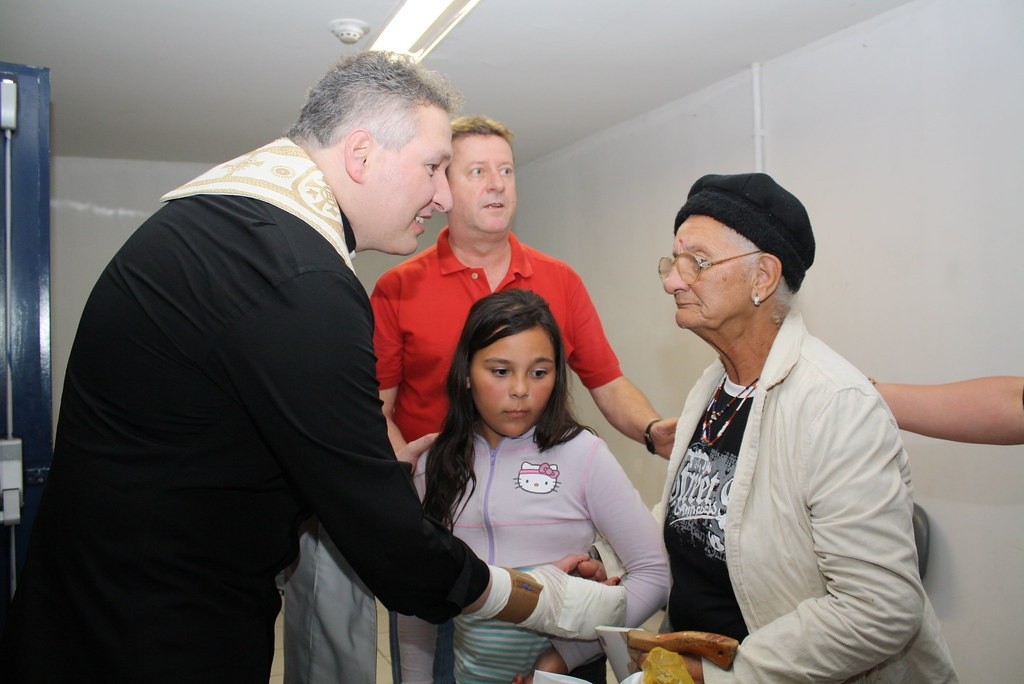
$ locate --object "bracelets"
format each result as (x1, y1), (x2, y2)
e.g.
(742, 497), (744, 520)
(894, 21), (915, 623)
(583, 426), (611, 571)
(644, 420), (660, 454)
(868, 377), (876, 385)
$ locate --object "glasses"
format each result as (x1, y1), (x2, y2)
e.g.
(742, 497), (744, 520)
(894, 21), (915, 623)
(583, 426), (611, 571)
(658, 250), (763, 285)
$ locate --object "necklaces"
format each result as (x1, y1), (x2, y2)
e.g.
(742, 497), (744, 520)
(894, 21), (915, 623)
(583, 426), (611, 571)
(700, 376), (760, 451)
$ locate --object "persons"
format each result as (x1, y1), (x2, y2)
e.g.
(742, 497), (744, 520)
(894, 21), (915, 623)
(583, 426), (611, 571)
(868, 375), (1024, 446)
(409, 287), (669, 684)
(367, 116), (679, 461)
(578, 173), (959, 684)
(0, 50), (627, 684)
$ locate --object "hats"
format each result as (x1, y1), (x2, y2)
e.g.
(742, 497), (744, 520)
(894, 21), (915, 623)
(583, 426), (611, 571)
(673, 172), (816, 294)
